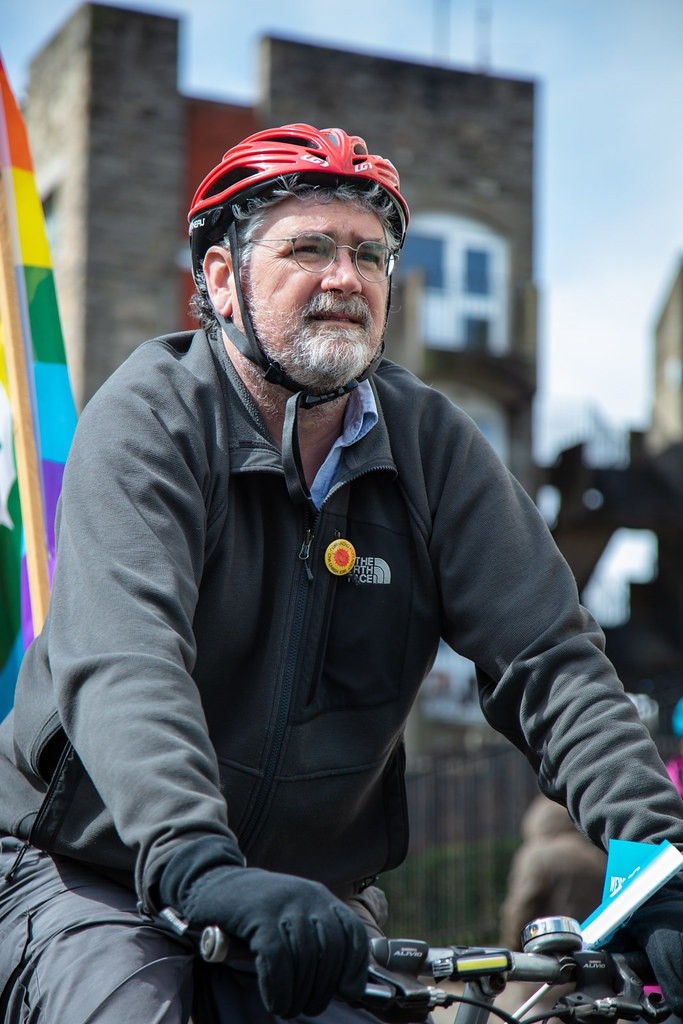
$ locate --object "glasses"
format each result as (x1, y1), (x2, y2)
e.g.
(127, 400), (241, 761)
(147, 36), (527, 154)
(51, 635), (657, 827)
(224, 232), (400, 283)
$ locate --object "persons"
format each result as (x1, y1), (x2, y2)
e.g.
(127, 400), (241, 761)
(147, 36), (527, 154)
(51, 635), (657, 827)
(0, 121), (682, 1023)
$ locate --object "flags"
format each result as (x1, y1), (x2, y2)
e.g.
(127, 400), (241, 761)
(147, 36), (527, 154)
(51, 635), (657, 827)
(0, 53), (81, 725)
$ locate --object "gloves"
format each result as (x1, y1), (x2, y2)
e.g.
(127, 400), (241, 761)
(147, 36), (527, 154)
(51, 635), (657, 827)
(162, 836), (368, 1021)
(622, 891), (683, 1024)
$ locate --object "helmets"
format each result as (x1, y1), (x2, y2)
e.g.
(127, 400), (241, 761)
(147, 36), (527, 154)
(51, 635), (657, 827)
(187, 123), (410, 298)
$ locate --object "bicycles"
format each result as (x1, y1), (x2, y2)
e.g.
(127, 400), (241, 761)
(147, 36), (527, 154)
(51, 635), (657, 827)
(198, 914), (674, 1023)
